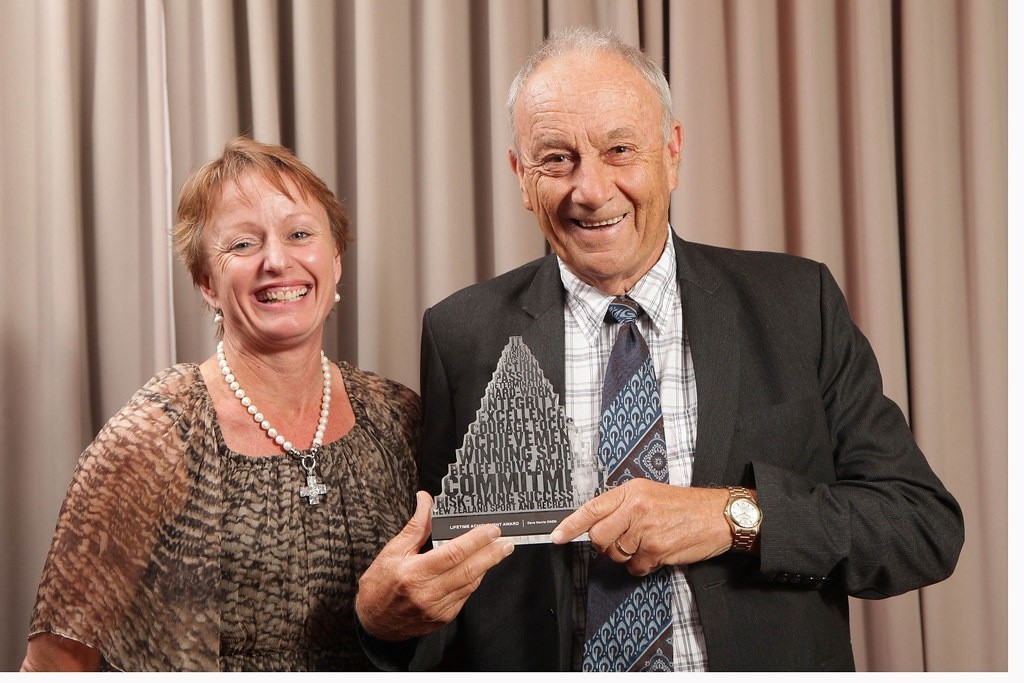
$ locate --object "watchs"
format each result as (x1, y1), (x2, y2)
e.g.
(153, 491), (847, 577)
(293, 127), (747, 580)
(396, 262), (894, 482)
(723, 484), (764, 552)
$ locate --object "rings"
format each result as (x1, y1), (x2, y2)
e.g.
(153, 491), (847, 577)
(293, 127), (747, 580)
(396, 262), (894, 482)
(615, 538), (633, 557)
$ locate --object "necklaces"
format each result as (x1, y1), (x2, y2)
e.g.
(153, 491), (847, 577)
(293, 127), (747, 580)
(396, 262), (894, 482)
(217, 341), (332, 505)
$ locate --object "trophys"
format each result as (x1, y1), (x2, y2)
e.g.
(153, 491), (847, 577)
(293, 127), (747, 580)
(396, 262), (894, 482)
(430, 337), (602, 550)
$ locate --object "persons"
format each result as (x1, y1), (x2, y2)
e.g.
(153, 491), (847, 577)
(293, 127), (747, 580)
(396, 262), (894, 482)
(17, 137), (422, 672)
(291, 28), (966, 672)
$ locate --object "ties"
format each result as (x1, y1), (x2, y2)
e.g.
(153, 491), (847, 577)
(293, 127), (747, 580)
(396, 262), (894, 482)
(581, 296), (674, 673)
